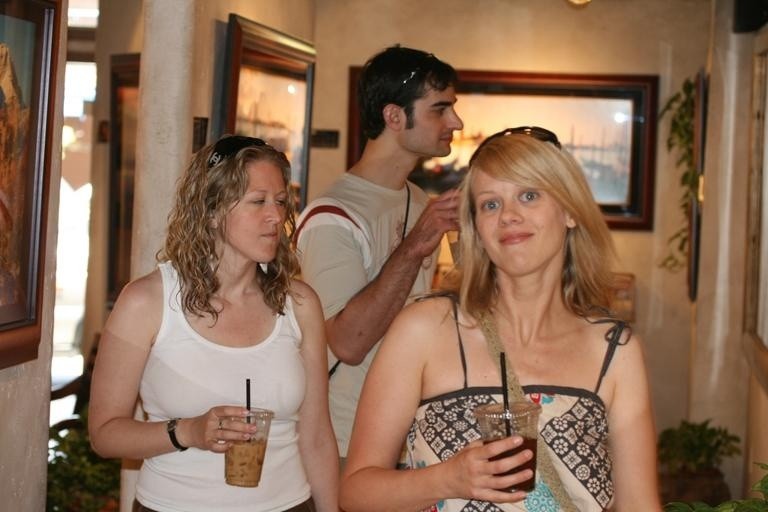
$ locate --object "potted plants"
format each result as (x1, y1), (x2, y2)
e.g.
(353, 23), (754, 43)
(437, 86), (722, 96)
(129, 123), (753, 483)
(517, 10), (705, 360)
(658, 417), (742, 507)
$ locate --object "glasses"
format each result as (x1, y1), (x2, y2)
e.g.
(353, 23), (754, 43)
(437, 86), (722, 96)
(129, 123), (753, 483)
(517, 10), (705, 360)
(205, 137), (265, 168)
(468, 126), (561, 169)
(387, 52), (432, 98)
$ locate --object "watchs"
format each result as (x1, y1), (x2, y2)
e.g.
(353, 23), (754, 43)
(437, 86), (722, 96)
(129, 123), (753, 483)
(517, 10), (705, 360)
(167, 418), (188, 451)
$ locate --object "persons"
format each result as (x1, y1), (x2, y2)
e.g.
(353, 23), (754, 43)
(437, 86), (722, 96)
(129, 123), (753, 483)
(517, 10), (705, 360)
(291, 44), (463, 470)
(88, 134), (340, 512)
(338, 125), (662, 512)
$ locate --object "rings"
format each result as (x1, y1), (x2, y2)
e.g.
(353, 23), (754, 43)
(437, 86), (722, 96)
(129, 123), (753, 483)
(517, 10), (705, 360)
(218, 420), (222, 429)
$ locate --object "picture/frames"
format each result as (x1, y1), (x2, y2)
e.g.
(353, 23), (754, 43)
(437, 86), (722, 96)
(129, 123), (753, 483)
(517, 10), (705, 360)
(738, 28), (768, 396)
(0, 0), (63, 369)
(345, 65), (660, 231)
(217, 12), (318, 214)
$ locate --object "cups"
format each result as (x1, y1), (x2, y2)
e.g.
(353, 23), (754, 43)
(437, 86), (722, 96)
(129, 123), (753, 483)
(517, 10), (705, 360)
(473, 402), (542, 492)
(224, 405), (275, 488)
